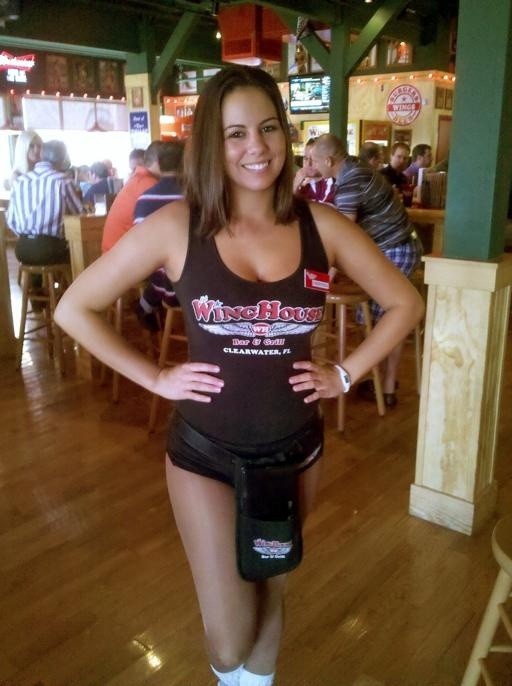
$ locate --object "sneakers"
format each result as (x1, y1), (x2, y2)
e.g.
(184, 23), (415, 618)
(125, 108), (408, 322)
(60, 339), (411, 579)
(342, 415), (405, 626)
(355, 380), (377, 401)
(131, 298), (160, 334)
(382, 393), (397, 406)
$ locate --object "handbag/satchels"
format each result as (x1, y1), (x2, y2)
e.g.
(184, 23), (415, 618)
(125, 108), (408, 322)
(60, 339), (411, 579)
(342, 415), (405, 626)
(232, 461), (303, 584)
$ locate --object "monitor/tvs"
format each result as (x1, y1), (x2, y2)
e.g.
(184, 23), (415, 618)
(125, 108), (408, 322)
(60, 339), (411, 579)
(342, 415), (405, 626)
(289, 72), (329, 114)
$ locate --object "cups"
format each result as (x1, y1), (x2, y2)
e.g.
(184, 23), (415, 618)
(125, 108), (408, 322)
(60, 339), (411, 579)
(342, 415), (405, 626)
(400, 182), (414, 207)
(93, 191), (106, 216)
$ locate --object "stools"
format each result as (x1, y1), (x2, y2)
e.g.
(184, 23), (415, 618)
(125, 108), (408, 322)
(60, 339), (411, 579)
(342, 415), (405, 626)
(459, 514), (511, 686)
(311, 283), (385, 433)
(148, 303), (186, 434)
(99, 278), (161, 403)
(411, 269), (426, 398)
(16, 263), (80, 378)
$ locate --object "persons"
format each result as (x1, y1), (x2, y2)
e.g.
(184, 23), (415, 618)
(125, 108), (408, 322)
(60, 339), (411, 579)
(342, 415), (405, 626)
(361, 141), (447, 208)
(6, 139), (86, 313)
(135, 141), (186, 308)
(293, 136), (337, 204)
(311, 135), (423, 411)
(51, 62), (429, 686)
(61, 154), (118, 213)
(9, 131), (43, 186)
(129, 149), (145, 172)
(100, 142), (161, 334)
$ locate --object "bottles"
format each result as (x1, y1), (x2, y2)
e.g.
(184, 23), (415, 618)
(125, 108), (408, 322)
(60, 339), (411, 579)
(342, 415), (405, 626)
(175, 106), (194, 119)
(294, 43), (307, 73)
(107, 176), (122, 195)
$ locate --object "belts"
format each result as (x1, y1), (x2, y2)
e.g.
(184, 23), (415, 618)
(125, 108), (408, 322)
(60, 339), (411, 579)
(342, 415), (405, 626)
(20, 234), (66, 243)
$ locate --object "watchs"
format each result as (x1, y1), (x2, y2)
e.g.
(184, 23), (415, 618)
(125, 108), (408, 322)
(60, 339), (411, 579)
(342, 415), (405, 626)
(334, 365), (351, 393)
